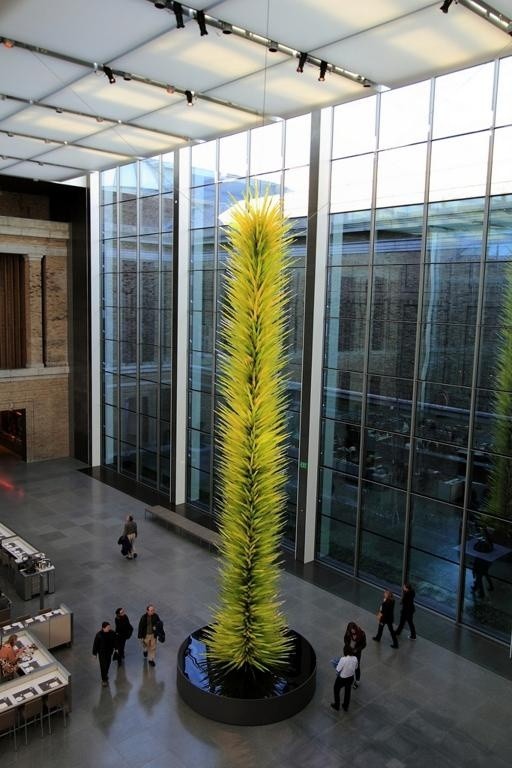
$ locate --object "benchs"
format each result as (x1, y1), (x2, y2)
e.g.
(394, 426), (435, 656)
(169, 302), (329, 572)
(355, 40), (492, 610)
(144, 505), (225, 563)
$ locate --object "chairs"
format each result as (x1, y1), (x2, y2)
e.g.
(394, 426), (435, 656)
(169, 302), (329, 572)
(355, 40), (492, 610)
(20, 697), (45, 745)
(45, 687), (67, 737)
(0, 709), (19, 753)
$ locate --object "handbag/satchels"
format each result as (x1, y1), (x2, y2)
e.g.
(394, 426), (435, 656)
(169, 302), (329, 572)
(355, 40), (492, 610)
(112, 649), (119, 661)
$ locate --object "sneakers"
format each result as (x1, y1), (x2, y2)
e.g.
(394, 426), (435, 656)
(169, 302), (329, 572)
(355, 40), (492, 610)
(353, 680), (361, 690)
(102, 681), (107, 687)
(408, 635), (417, 641)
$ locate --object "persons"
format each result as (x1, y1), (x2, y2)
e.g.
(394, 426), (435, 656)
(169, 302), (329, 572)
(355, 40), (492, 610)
(112, 607), (134, 666)
(92, 621), (118, 687)
(137, 605), (165, 667)
(330, 645), (359, 713)
(122, 515), (137, 560)
(396, 583), (417, 639)
(372, 590), (399, 649)
(0, 634), (24, 674)
(343, 621), (367, 690)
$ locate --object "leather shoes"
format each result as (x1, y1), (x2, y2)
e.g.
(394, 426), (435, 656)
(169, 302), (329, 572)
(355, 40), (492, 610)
(330, 703), (340, 710)
(342, 703), (348, 711)
(372, 636), (381, 642)
(148, 660), (155, 667)
(143, 651), (148, 657)
(390, 644), (398, 649)
